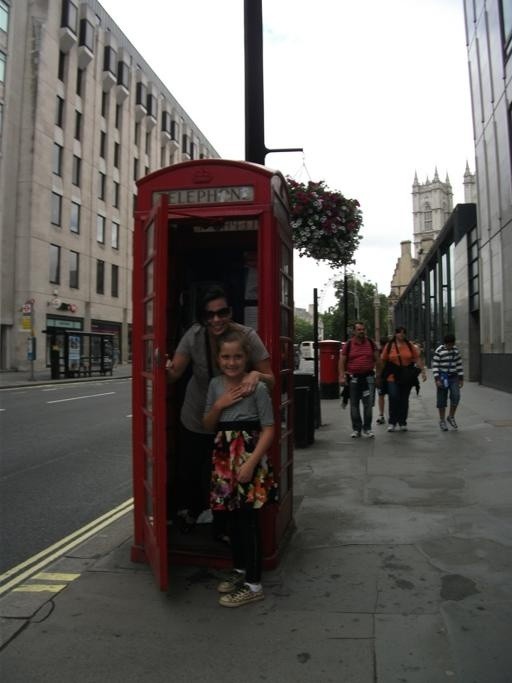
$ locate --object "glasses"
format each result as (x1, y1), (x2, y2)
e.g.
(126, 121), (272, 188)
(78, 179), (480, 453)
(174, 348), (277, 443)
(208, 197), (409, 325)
(206, 307), (228, 320)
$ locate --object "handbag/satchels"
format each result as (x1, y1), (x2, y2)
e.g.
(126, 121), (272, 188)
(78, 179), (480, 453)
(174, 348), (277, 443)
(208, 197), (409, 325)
(440, 370), (449, 388)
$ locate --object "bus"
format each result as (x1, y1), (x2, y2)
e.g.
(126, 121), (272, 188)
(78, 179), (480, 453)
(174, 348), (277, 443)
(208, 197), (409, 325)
(300, 340), (319, 361)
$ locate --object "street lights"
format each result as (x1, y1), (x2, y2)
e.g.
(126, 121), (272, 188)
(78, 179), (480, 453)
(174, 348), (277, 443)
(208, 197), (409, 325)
(338, 287), (360, 320)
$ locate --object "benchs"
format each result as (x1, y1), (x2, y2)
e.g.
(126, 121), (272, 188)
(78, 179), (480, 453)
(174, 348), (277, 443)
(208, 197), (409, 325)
(58, 369), (111, 378)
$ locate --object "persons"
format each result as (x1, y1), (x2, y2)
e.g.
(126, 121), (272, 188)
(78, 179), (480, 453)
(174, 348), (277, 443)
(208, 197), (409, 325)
(338, 321), (380, 440)
(204, 335), (275, 608)
(155, 295), (275, 543)
(374, 327), (427, 433)
(431, 333), (465, 431)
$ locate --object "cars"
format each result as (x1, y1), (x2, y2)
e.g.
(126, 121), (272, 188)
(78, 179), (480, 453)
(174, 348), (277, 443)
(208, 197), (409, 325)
(293, 343), (300, 369)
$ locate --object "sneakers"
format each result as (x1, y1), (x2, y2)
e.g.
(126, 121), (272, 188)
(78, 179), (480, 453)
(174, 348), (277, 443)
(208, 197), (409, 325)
(363, 430), (374, 438)
(218, 569), (244, 591)
(219, 585), (264, 607)
(439, 420), (447, 431)
(376, 416), (385, 423)
(387, 424), (394, 431)
(447, 416), (457, 428)
(352, 431), (361, 437)
(400, 426), (406, 430)
